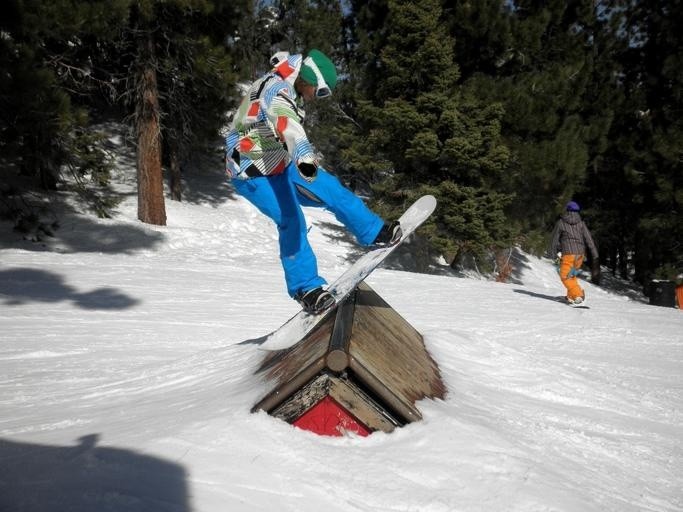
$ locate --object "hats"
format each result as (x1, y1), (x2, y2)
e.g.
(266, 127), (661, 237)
(301, 48), (337, 92)
(567, 201), (580, 211)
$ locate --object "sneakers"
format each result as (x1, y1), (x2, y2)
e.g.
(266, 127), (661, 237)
(372, 222), (403, 246)
(574, 296), (583, 304)
(295, 287), (335, 311)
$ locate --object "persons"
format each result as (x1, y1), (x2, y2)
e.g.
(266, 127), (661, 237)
(551, 200), (598, 304)
(224, 49), (404, 317)
(672, 254), (683, 311)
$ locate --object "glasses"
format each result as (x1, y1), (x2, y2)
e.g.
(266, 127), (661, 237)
(302, 56), (333, 99)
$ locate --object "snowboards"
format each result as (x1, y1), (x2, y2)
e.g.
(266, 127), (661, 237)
(556, 296), (589, 308)
(257, 194), (437, 351)
